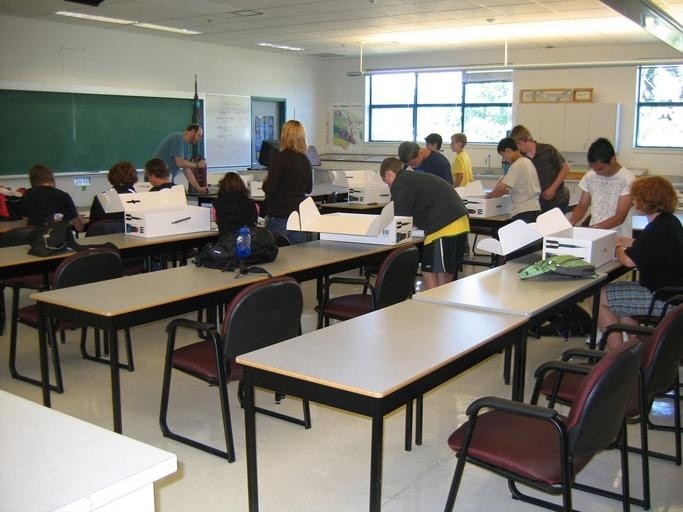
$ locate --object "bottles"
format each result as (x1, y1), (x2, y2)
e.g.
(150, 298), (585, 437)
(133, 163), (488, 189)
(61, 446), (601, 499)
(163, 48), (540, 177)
(235, 225), (251, 262)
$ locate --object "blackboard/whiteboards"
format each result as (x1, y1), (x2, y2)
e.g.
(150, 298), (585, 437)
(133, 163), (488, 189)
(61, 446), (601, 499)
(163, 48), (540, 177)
(0, 88), (203, 179)
(205, 95), (252, 165)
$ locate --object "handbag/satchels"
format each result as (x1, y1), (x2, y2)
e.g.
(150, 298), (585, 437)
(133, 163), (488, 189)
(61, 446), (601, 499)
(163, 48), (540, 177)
(27, 213), (79, 258)
(0, 185), (25, 221)
(517, 253), (595, 280)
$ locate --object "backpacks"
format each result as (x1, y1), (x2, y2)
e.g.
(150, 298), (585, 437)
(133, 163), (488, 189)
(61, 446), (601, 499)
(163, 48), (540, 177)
(192, 224), (278, 281)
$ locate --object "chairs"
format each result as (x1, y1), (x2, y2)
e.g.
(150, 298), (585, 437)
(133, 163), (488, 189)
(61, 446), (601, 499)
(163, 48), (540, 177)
(539, 286), (683, 510)
(442, 338), (647, 510)
(159, 278), (311, 464)
(7, 247), (134, 394)
(315, 244), (420, 331)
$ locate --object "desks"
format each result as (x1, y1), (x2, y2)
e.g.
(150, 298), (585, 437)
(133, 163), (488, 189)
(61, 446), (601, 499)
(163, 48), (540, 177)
(30, 233), (426, 436)
(2, 208), (265, 335)
(302, 200), (576, 267)
(234, 249), (636, 512)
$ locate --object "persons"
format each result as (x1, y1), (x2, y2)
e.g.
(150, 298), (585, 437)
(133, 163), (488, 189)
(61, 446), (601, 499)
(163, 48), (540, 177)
(586, 174), (682, 352)
(89, 161), (139, 222)
(379, 157), (470, 289)
(397, 140), (453, 187)
(144, 123), (208, 198)
(209, 171), (261, 241)
(423, 132), (443, 153)
(17, 163), (87, 233)
(447, 132), (473, 188)
(142, 158), (186, 192)
(260, 119), (313, 238)
(509, 124), (570, 216)
(484, 136), (543, 262)
(566, 137), (638, 240)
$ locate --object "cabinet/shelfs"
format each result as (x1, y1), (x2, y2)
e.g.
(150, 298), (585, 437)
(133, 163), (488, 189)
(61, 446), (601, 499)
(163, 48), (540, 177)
(564, 103), (624, 155)
(515, 101), (565, 154)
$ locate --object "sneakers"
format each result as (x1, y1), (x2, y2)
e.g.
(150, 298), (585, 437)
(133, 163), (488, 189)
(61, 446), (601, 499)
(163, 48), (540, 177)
(587, 331), (603, 342)
